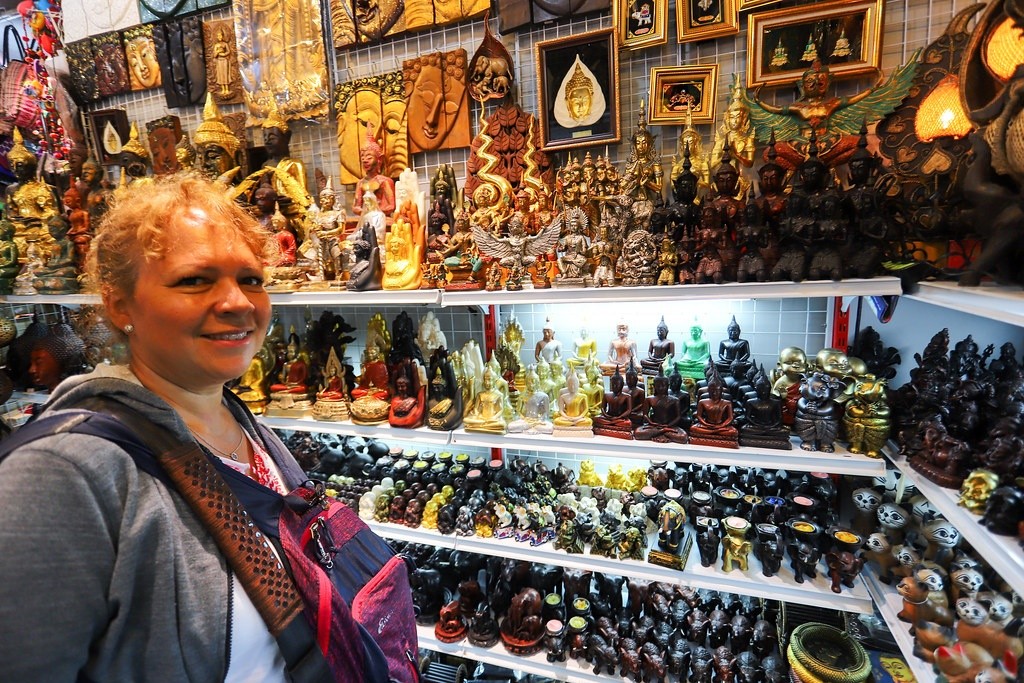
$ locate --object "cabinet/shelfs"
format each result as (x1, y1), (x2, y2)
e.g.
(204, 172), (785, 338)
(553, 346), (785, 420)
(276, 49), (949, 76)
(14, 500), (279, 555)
(0, 275), (1024, 683)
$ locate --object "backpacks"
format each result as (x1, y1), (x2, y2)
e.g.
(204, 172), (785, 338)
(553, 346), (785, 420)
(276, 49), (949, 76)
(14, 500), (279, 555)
(0, 412), (420, 682)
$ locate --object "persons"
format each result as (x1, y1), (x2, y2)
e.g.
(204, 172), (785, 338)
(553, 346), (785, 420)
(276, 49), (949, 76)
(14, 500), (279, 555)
(565, 62), (594, 121)
(315, 48), (889, 292)
(229, 310), (868, 452)
(0, 13), (346, 296)
(325, 475), (497, 538)
(0, 174), (314, 683)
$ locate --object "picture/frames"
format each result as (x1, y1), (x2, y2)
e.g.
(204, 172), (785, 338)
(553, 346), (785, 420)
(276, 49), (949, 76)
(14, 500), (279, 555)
(648, 63), (720, 125)
(612, 0), (669, 53)
(745, 0), (887, 92)
(675, 0), (740, 44)
(533, 25), (623, 153)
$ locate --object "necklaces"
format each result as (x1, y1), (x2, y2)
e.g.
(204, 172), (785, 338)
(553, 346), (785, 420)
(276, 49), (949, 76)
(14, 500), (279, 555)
(185, 424), (244, 461)
